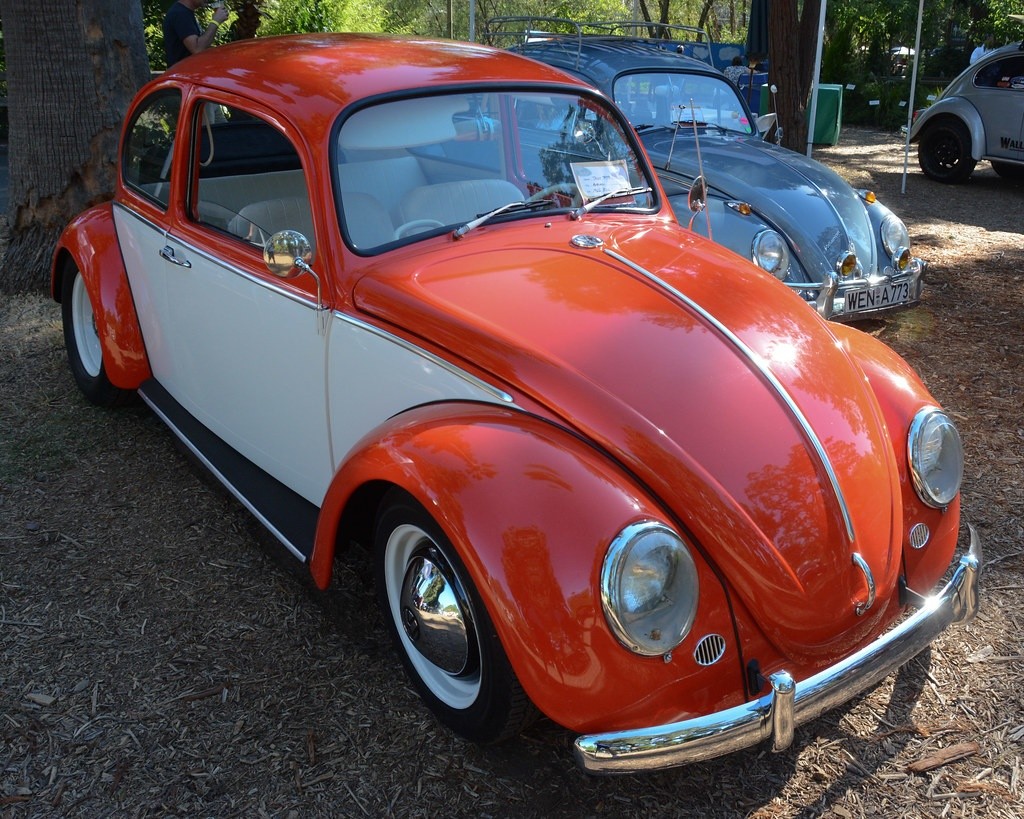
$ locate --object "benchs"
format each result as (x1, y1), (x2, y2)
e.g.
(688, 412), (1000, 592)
(140, 156), (427, 226)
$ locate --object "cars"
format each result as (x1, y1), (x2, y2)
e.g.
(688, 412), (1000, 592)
(47, 32), (987, 781)
(401, 15), (930, 324)
(885, 37), (1024, 185)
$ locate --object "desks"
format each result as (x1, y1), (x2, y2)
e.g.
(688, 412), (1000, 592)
(653, 108), (737, 121)
(760, 82), (843, 147)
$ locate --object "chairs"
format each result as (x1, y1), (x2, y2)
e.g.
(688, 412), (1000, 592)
(399, 179), (526, 236)
(228, 193), (394, 250)
(755, 113), (777, 139)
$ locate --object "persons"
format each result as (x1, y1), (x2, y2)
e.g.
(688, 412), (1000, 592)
(675, 45), (685, 54)
(970, 36), (996, 64)
(724, 57), (759, 87)
